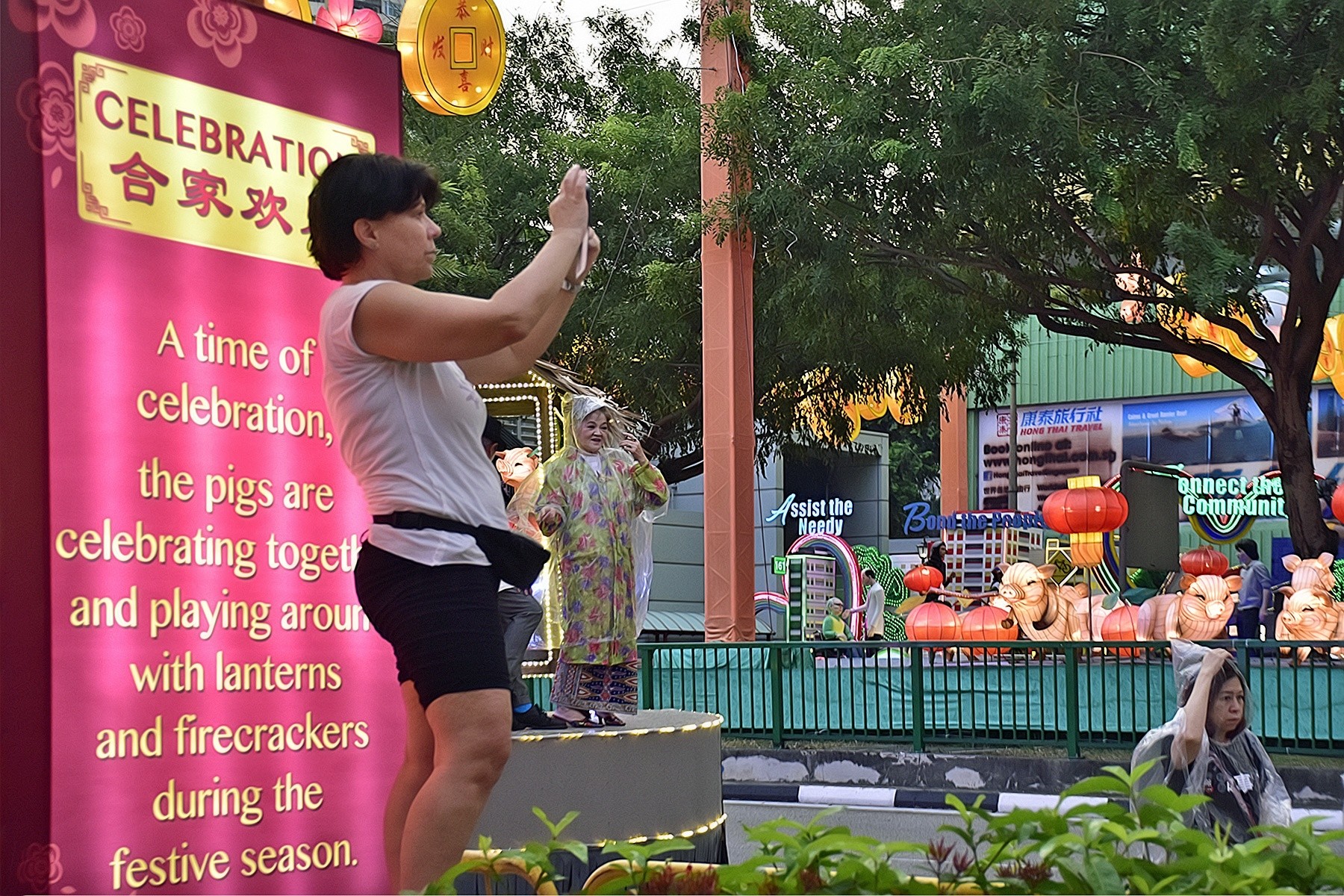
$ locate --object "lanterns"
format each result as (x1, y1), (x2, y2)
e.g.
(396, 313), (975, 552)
(1101, 604), (1154, 658)
(904, 567), (943, 596)
(1181, 546), (1228, 576)
(1043, 475), (1129, 569)
(961, 605), (1018, 655)
(905, 601), (961, 659)
(1331, 484), (1344, 524)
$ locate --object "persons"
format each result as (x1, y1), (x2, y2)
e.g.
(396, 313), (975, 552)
(918, 541), (956, 609)
(1234, 539), (1271, 637)
(308, 154), (601, 895)
(480, 413), (568, 730)
(822, 598), (864, 657)
(842, 568), (884, 658)
(1131, 639), (1291, 864)
(535, 393), (669, 729)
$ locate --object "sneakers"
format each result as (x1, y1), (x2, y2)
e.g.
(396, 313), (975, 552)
(512, 704), (567, 731)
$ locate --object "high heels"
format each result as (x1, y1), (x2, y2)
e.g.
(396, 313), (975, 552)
(553, 713), (603, 727)
(595, 711), (626, 726)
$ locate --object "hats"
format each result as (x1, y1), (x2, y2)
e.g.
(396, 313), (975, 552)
(482, 416), (506, 451)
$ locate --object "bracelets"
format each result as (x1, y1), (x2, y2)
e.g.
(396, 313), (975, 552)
(561, 278), (581, 294)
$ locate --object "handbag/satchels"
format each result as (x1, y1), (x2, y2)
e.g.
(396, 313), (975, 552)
(372, 509), (550, 589)
